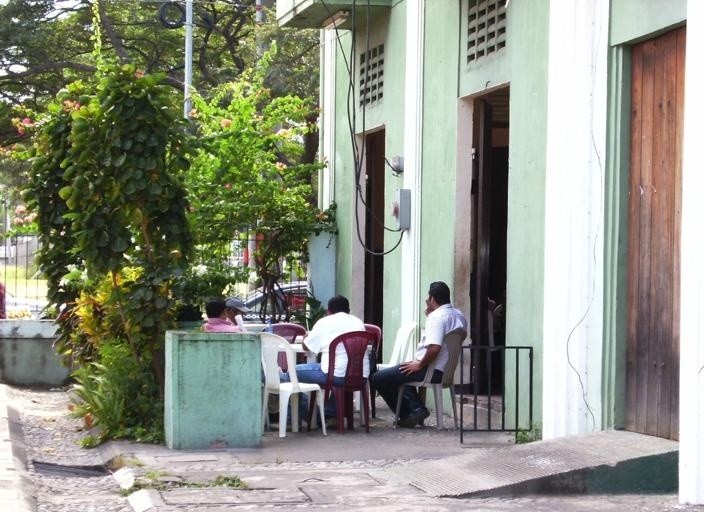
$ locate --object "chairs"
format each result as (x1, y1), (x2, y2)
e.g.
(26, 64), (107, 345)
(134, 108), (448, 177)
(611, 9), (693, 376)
(239, 320), (466, 438)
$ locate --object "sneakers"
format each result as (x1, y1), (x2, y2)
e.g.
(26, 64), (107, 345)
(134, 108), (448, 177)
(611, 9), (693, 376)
(402, 408), (430, 427)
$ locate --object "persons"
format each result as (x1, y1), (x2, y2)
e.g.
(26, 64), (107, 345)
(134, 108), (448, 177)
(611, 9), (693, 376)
(287, 294), (371, 431)
(199, 297), (287, 423)
(372, 281), (467, 428)
(225, 298), (253, 327)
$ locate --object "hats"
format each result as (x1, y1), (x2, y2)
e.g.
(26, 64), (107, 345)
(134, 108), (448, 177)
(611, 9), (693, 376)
(224, 297), (252, 314)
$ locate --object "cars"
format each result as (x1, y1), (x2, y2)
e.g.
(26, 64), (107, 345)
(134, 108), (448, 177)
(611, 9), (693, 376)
(233, 281), (307, 320)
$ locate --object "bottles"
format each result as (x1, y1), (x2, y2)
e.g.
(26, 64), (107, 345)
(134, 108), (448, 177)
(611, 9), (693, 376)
(266, 320), (273, 334)
